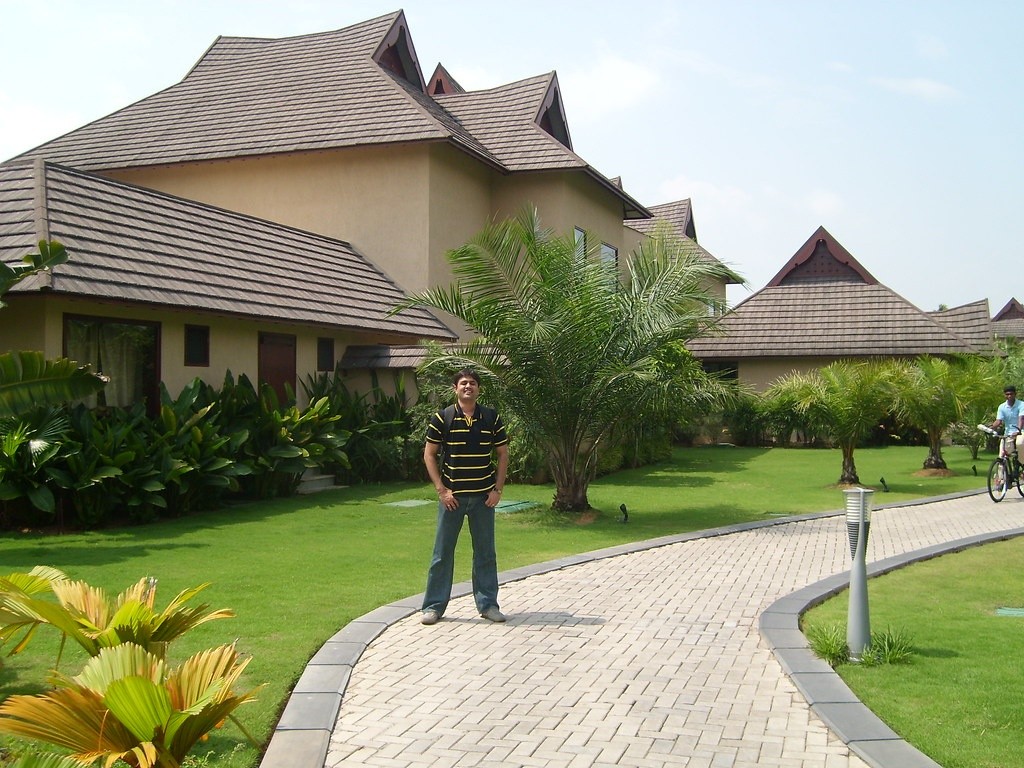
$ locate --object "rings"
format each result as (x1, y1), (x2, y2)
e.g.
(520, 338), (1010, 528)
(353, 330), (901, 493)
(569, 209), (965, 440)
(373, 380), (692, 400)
(492, 504), (494, 506)
(447, 505), (450, 506)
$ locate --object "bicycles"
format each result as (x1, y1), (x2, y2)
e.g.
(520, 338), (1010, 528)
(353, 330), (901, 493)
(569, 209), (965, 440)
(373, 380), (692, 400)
(985, 424), (1024, 503)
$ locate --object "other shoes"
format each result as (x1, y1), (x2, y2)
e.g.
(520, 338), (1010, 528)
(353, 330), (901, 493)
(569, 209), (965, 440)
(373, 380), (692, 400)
(482, 606), (506, 622)
(421, 611), (438, 624)
(994, 480), (1001, 491)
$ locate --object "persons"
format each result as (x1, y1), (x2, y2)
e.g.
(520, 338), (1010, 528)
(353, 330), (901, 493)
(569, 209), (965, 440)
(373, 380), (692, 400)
(420, 368), (511, 624)
(985, 386), (1024, 490)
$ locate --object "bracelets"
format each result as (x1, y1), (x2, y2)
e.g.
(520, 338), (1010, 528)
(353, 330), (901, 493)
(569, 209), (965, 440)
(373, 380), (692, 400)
(439, 489), (447, 494)
(493, 488), (502, 493)
